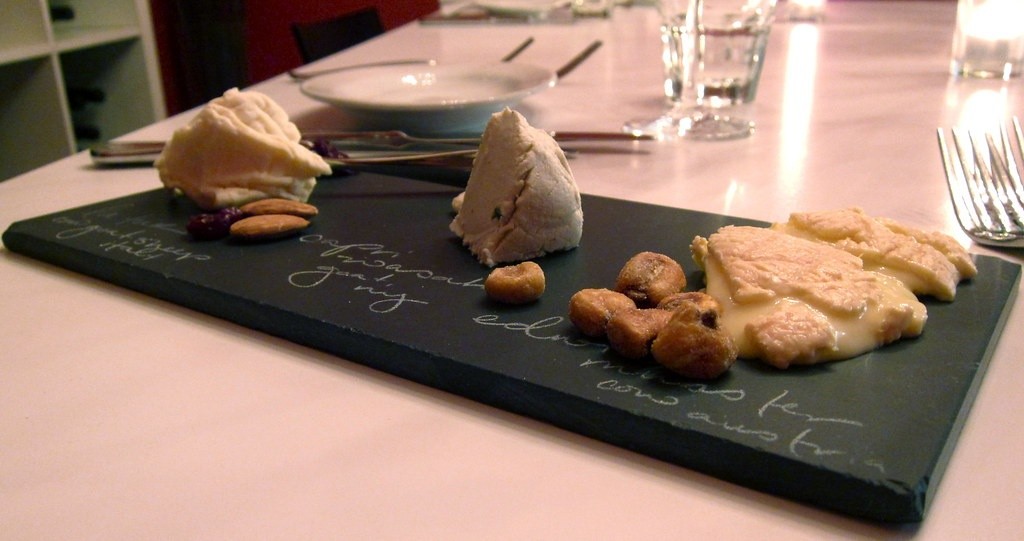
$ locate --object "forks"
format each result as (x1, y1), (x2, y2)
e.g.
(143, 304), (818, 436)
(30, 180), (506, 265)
(937, 115), (1024, 248)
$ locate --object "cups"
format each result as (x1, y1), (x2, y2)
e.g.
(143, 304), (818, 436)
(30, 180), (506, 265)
(949, 0), (1024, 81)
(654, 0), (775, 105)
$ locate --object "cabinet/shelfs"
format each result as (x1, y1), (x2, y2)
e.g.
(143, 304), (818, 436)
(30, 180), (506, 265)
(1, 1), (171, 185)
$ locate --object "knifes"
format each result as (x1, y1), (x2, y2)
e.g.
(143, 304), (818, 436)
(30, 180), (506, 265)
(89, 129), (652, 162)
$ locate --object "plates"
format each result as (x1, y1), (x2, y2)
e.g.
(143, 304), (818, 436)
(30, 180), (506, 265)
(1, 164), (1023, 528)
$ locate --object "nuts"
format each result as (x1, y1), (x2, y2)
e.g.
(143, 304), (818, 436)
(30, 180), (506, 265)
(224, 197), (317, 242)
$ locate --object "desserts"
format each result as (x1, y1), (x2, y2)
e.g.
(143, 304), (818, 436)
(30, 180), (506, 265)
(153, 87), (332, 211)
(689, 207), (979, 371)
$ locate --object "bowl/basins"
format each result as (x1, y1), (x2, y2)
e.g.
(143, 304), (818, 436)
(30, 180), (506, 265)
(300, 58), (557, 132)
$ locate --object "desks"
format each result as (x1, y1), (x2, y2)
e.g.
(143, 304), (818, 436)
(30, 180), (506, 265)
(0, 0), (1022, 541)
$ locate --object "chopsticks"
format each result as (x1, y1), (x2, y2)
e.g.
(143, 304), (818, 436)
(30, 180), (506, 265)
(501, 36), (602, 78)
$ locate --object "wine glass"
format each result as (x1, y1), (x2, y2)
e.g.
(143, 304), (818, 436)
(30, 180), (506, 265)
(621, 0), (755, 143)
